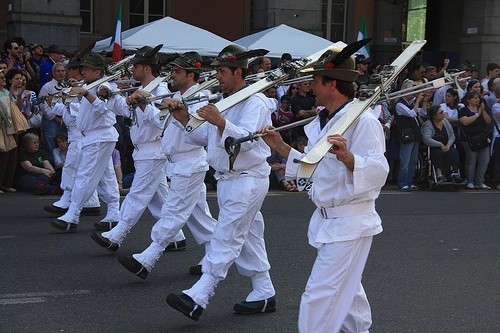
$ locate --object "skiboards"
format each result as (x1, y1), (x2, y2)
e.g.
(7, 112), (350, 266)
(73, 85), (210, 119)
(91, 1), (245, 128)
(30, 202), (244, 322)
(154, 56), (260, 124)
(184, 40), (348, 136)
(47, 53), (136, 111)
(293, 40), (428, 192)
(129, 67), (172, 99)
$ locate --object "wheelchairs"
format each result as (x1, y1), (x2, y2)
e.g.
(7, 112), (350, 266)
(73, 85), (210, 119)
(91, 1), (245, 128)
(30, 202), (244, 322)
(417, 144), (464, 190)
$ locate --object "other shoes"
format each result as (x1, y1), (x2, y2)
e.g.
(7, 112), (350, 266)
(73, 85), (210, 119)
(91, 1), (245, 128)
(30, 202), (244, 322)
(400, 171), (500, 192)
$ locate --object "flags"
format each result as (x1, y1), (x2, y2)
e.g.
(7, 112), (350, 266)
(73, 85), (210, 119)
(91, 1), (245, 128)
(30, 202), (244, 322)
(110, 0), (122, 61)
(355, 16), (369, 58)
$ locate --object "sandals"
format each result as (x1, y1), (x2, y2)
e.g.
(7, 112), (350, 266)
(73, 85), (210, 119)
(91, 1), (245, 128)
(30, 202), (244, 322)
(285, 183), (299, 191)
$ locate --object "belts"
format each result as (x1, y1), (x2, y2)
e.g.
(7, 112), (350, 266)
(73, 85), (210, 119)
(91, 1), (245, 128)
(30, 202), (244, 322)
(219, 161), (267, 175)
(80, 128), (108, 137)
(167, 147), (204, 163)
(66, 126), (77, 132)
(320, 202), (375, 219)
(135, 141), (161, 151)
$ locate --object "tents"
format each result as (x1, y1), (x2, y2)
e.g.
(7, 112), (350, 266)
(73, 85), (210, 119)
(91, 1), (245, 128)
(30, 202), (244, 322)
(91, 16), (246, 57)
(232, 24), (335, 59)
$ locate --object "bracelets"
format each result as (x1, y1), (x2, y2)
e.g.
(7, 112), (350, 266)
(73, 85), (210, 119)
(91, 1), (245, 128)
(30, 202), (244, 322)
(84, 90), (89, 97)
(475, 113), (479, 117)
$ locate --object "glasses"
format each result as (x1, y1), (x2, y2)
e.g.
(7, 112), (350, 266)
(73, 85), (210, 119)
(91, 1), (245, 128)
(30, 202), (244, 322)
(11, 47), (19, 50)
(360, 62), (369, 65)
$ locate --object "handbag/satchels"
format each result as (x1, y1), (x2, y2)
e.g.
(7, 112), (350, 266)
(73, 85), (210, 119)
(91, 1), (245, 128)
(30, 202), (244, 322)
(399, 127), (414, 142)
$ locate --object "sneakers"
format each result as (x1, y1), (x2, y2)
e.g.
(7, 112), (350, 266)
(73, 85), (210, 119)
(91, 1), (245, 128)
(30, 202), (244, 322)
(234, 295), (276, 314)
(117, 255), (148, 280)
(166, 292), (203, 322)
(189, 264), (202, 275)
(43, 203), (119, 254)
(163, 239), (186, 252)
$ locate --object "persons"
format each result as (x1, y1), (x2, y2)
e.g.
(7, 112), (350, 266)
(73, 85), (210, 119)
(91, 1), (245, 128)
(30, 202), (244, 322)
(0, 37), (500, 333)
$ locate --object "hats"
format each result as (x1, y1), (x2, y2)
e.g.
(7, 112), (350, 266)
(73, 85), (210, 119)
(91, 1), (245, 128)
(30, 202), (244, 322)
(30, 42), (63, 53)
(134, 46), (158, 64)
(170, 52), (204, 69)
(299, 37), (374, 82)
(210, 45), (270, 68)
(355, 55), (369, 64)
(64, 41), (105, 70)
